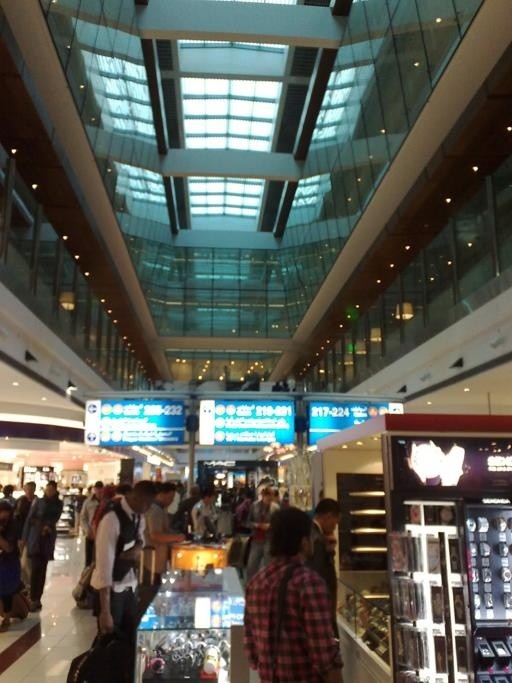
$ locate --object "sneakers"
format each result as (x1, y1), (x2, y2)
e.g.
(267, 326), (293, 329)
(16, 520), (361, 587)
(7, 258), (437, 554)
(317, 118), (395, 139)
(30, 605), (40, 611)
(0, 616), (10, 631)
(12, 615), (28, 623)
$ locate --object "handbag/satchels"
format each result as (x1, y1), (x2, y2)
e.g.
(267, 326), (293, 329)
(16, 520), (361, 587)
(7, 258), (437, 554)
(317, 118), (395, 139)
(74, 563), (97, 609)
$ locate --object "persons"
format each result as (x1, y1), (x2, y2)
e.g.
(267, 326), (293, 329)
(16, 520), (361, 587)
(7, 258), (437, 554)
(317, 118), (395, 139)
(222, 477), (344, 682)
(180, 479), (220, 539)
(75, 479), (184, 682)
(0, 479), (64, 620)
